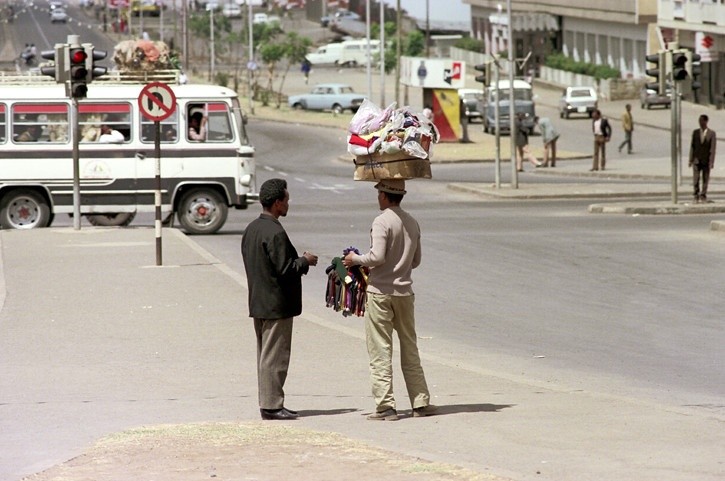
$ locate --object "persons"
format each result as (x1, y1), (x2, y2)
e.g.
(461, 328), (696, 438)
(81, 117), (125, 143)
(241, 178), (319, 420)
(188, 112), (209, 142)
(688, 115), (716, 204)
(513, 112), (530, 172)
(617, 104), (636, 154)
(344, 177), (441, 421)
(300, 58), (311, 86)
(16, 125), (41, 142)
(532, 116), (560, 168)
(589, 109), (611, 172)
(20, 43), (37, 65)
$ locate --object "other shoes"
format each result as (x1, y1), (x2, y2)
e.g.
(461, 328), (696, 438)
(367, 409), (399, 421)
(259, 406), (298, 420)
(412, 406), (440, 417)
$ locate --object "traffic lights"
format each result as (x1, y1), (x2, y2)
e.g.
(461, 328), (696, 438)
(474, 61), (491, 87)
(669, 48), (691, 83)
(688, 52), (703, 90)
(65, 46), (89, 100)
(81, 43), (108, 84)
(39, 43), (65, 83)
(644, 51), (667, 96)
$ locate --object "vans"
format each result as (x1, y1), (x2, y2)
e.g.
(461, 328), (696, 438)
(303, 38), (384, 68)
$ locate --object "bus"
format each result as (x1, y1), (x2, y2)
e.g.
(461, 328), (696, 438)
(0, 67), (259, 235)
(482, 79), (537, 136)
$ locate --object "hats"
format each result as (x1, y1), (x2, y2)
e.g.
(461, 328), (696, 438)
(373, 178), (407, 194)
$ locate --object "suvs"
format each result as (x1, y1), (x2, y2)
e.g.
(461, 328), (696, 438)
(558, 86), (599, 120)
(459, 87), (486, 122)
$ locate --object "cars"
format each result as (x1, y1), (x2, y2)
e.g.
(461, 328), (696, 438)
(640, 82), (673, 110)
(48, 1), (67, 24)
(130, 0), (161, 18)
(288, 83), (366, 115)
(202, 1), (269, 28)
